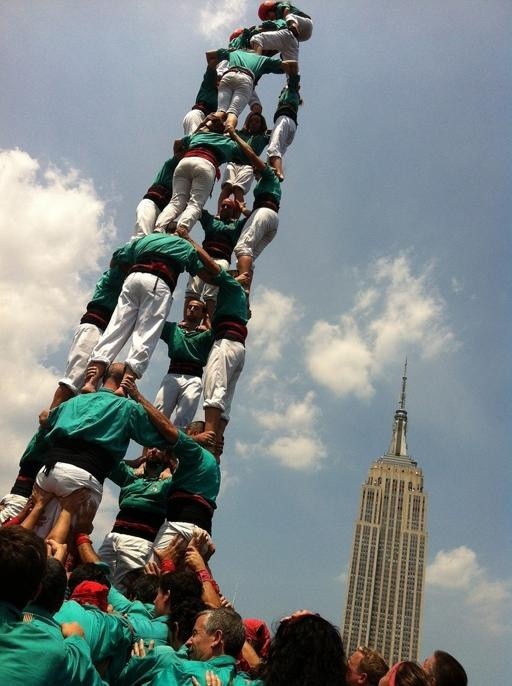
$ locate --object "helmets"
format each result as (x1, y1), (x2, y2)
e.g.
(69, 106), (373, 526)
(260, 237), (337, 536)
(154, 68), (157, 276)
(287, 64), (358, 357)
(257, 0), (277, 21)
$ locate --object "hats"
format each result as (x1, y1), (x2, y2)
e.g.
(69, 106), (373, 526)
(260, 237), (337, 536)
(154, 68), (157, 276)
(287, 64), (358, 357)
(67, 578), (110, 613)
